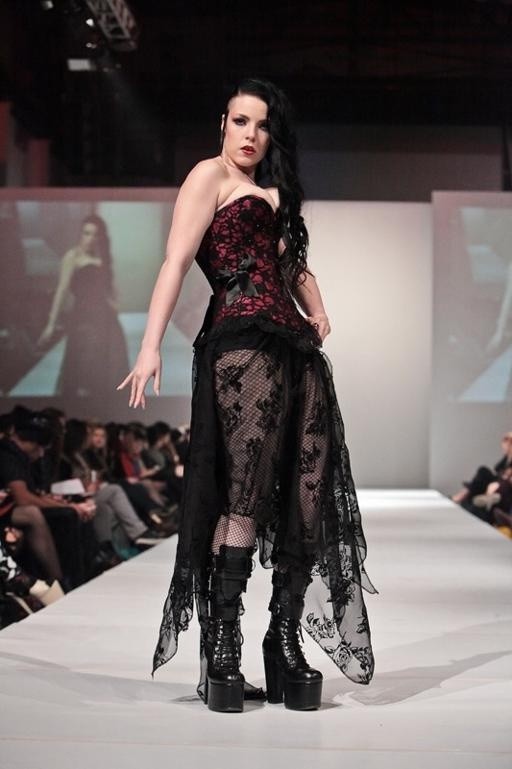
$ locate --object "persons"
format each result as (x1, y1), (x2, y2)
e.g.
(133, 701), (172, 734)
(115, 79), (380, 714)
(452, 432), (511, 527)
(1, 402), (194, 634)
(37, 211), (134, 398)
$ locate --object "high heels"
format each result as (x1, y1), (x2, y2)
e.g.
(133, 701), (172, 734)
(261, 601), (326, 711)
(196, 603), (245, 714)
